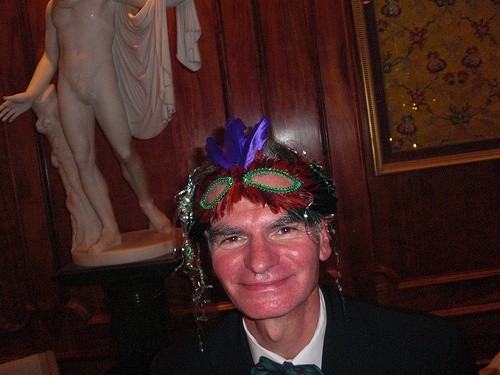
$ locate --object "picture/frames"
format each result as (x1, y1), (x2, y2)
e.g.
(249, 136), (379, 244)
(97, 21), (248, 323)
(348, 0), (500, 177)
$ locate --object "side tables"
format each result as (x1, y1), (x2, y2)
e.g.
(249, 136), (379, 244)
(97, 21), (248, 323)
(45, 245), (185, 375)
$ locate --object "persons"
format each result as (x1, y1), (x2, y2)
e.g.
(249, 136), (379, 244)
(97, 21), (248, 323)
(1, 0), (191, 258)
(147, 116), (479, 375)
